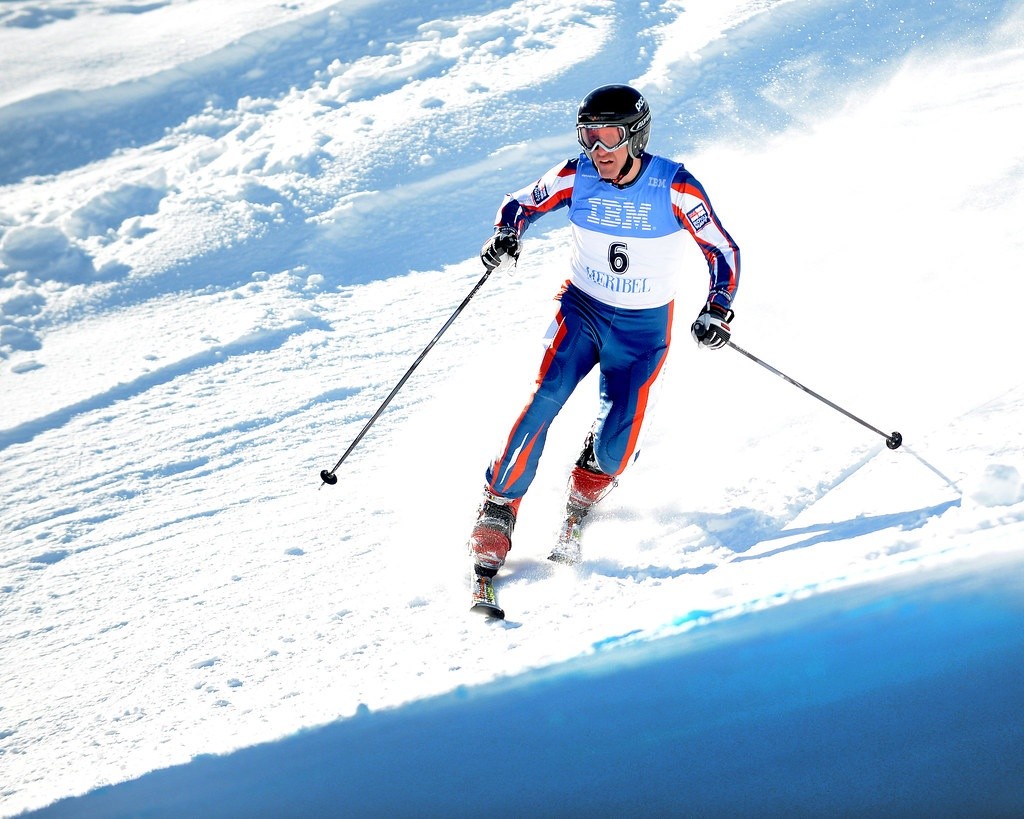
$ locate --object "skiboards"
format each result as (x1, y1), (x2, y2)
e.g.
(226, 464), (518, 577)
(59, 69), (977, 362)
(466, 501), (589, 620)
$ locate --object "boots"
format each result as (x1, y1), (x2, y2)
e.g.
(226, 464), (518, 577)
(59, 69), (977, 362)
(547, 466), (615, 565)
(466, 482), (522, 582)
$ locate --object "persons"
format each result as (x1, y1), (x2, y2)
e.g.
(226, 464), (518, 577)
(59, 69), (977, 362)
(467, 84), (741, 578)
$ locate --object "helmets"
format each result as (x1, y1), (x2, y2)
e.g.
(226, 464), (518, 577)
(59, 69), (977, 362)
(576, 84), (651, 158)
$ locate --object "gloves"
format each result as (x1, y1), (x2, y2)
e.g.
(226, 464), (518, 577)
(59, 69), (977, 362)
(691, 301), (734, 350)
(480, 226), (521, 271)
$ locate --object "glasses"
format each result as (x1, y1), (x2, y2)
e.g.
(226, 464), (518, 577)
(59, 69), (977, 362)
(577, 122), (631, 152)
